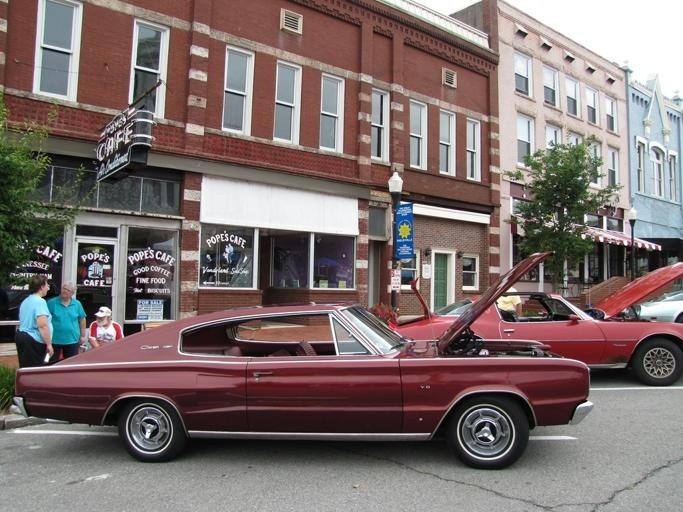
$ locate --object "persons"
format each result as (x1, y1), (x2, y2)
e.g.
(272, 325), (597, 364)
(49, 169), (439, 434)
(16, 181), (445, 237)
(495, 287), (522, 322)
(46, 282), (87, 364)
(15, 275), (53, 417)
(87, 306), (124, 349)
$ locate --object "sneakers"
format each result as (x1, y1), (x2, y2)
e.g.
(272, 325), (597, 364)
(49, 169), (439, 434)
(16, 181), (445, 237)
(9, 404), (22, 414)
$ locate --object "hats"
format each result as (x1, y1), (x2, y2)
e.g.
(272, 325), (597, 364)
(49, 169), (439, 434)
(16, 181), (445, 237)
(93, 305), (112, 318)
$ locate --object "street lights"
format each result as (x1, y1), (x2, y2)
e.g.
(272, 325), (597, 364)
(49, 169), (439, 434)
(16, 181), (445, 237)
(628, 207), (637, 280)
(388, 171), (403, 307)
